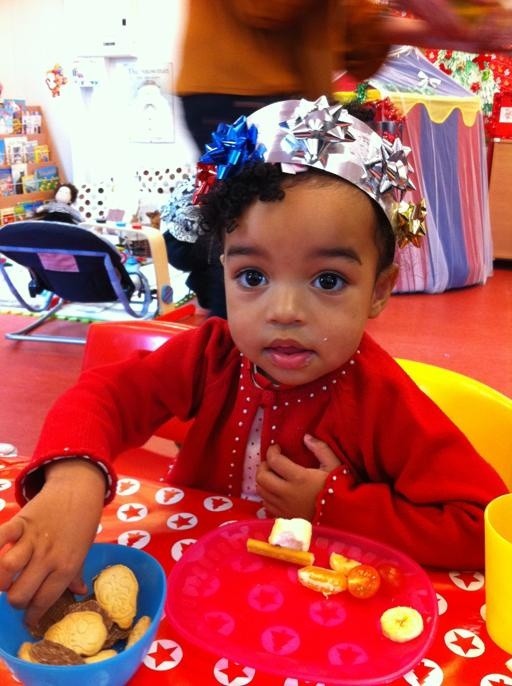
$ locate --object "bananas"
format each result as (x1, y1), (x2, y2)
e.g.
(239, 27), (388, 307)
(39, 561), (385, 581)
(380, 606), (424, 643)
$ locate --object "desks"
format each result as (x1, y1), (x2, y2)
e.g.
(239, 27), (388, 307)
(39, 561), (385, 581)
(0, 455), (512, 686)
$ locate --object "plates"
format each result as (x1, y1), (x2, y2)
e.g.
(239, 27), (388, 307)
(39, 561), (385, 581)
(163, 515), (436, 686)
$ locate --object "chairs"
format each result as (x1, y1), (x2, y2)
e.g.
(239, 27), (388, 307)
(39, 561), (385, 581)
(0, 220), (154, 346)
(393, 356), (512, 491)
(81, 321), (196, 483)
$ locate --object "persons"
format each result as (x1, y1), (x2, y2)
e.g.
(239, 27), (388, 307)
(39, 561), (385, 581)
(31, 180), (83, 224)
(168, 0), (512, 158)
(2, 91), (512, 633)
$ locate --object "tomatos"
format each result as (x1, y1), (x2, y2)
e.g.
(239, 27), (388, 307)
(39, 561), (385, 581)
(347, 565), (381, 599)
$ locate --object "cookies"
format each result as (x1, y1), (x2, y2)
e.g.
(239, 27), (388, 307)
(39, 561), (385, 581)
(18, 564), (151, 666)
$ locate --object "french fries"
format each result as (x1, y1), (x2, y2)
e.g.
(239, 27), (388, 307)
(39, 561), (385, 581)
(247, 539), (315, 568)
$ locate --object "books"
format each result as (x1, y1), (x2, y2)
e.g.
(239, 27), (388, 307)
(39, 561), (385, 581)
(0, 98), (59, 195)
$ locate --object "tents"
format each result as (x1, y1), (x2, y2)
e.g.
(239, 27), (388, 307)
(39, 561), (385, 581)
(329, 44), (494, 295)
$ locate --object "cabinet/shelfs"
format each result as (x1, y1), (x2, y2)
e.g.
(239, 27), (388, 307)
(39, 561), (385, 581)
(0, 105), (67, 228)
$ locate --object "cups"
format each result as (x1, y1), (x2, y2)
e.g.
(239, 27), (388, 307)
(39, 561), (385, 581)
(0, 541), (166, 686)
(482, 493), (512, 659)
